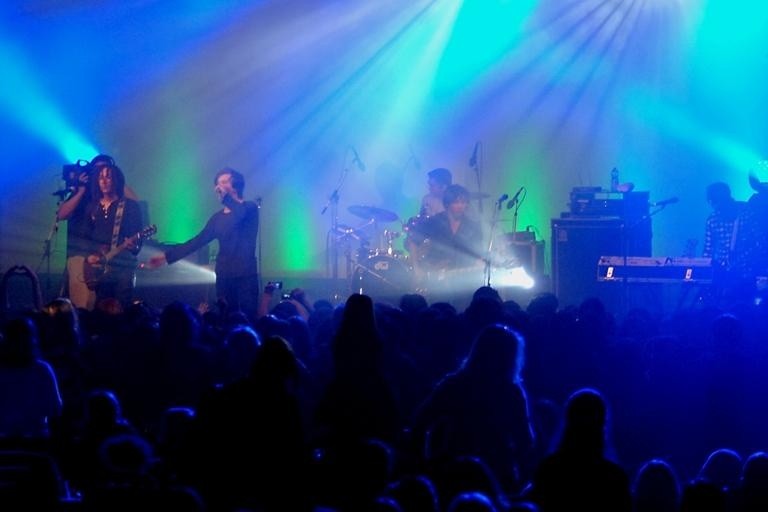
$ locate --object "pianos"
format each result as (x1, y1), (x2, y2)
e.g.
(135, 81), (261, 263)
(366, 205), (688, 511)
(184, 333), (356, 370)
(597, 255), (714, 285)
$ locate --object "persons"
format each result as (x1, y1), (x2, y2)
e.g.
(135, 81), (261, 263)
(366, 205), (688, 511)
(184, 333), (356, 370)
(148, 168), (261, 320)
(80, 164), (144, 310)
(404, 184), (485, 271)
(419, 168), (453, 217)
(702, 180), (767, 303)
(2, 281), (768, 512)
(58, 154), (139, 314)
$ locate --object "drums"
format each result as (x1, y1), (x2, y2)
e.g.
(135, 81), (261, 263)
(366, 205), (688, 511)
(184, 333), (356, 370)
(350, 254), (409, 297)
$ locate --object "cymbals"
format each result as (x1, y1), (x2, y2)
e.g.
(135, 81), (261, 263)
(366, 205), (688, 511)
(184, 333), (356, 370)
(331, 224), (366, 238)
(346, 205), (397, 223)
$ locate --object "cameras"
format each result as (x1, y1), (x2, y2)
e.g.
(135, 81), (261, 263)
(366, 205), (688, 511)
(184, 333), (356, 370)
(271, 280), (282, 290)
(282, 291), (294, 300)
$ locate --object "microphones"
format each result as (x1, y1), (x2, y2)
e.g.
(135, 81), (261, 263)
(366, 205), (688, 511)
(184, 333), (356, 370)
(495, 194), (508, 205)
(353, 149), (366, 172)
(469, 144), (478, 167)
(506, 187), (522, 208)
(652, 198), (679, 206)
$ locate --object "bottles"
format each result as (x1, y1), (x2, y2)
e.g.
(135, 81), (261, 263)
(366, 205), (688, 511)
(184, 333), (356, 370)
(610, 167), (621, 192)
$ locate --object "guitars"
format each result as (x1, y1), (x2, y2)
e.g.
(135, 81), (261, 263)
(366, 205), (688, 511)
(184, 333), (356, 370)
(413, 259), (523, 295)
(83, 224), (157, 291)
